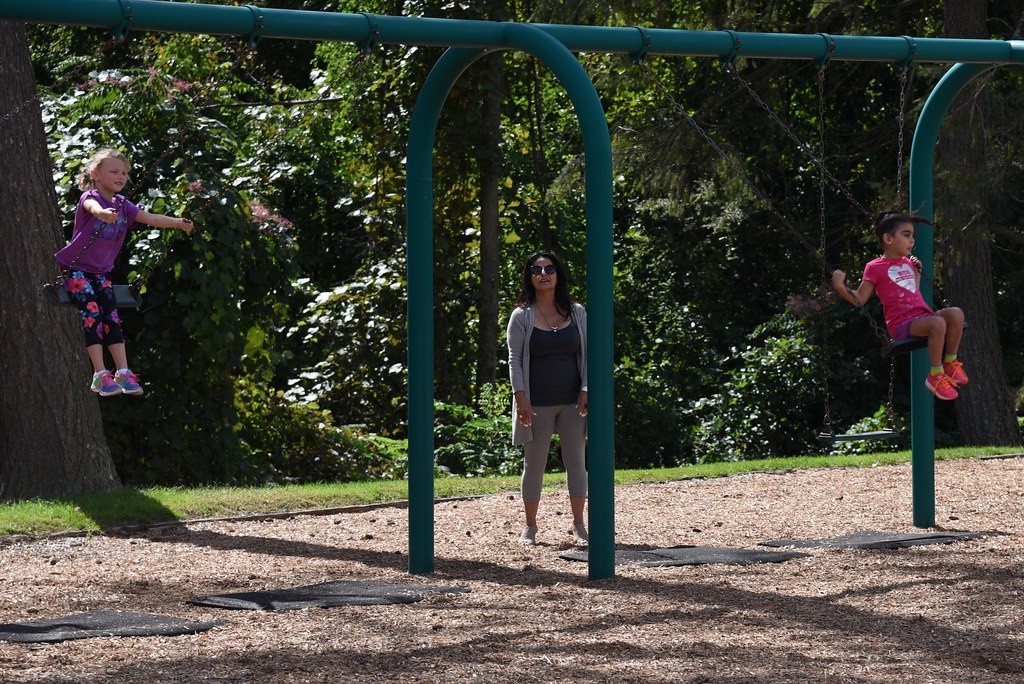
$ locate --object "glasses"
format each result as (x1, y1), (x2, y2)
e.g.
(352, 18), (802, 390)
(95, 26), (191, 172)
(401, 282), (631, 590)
(530, 265), (557, 276)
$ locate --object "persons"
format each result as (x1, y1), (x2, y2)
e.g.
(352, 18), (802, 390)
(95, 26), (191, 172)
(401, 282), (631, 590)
(53, 148), (193, 396)
(506, 249), (589, 545)
(832, 209), (968, 400)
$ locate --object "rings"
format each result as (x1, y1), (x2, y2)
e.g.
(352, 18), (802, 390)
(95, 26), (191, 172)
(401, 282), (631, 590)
(518, 414), (523, 419)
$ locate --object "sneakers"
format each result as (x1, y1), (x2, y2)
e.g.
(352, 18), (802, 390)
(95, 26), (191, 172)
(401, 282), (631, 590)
(943, 359), (968, 385)
(925, 372), (960, 400)
(91, 368), (122, 396)
(114, 370), (143, 395)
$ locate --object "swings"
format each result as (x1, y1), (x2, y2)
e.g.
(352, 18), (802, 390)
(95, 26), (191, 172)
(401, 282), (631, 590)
(33, 41), (374, 309)
(625, 57), (981, 364)
(811, 64), (916, 448)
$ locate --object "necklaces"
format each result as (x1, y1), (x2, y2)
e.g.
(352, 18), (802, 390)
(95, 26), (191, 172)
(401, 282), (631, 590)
(535, 302), (559, 332)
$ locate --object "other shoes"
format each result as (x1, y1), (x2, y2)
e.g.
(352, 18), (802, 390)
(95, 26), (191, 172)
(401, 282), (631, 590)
(518, 526), (537, 547)
(572, 520), (588, 546)
